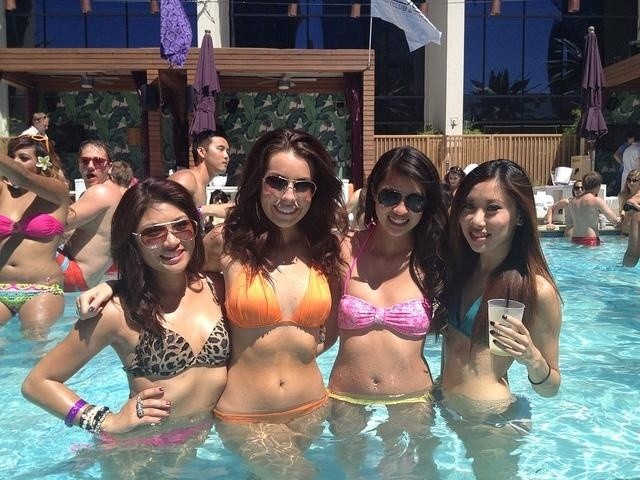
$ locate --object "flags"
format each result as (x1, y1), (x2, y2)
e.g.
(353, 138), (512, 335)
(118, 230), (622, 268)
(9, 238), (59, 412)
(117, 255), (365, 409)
(371, 1), (442, 54)
(157, 2), (193, 68)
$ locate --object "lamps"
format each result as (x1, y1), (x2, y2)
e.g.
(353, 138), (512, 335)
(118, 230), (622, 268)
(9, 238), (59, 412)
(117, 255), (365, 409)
(5, 1), (597, 20)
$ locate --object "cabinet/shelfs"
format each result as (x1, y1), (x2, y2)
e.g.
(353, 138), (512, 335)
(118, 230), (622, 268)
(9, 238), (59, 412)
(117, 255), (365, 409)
(533, 184), (607, 223)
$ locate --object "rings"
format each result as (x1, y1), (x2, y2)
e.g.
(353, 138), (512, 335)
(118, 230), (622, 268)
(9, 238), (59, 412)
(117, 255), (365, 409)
(135, 396), (144, 418)
(76, 306), (80, 315)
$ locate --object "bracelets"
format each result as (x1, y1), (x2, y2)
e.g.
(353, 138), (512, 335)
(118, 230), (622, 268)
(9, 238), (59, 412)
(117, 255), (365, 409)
(524, 363), (551, 386)
(64, 398), (111, 435)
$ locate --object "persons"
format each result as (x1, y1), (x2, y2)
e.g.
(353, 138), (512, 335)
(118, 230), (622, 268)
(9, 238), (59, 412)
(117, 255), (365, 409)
(324, 143), (438, 478)
(74, 126), (336, 479)
(542, 132), (639, 269)
(22, 176), (231, 479)
(431, 158), (565, 479)
(0, 135), (69, 370)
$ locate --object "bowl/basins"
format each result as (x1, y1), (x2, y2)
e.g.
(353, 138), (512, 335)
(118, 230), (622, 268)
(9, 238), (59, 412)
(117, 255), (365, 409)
(209, 172), (229, 188)
(548, 170), (575, 186)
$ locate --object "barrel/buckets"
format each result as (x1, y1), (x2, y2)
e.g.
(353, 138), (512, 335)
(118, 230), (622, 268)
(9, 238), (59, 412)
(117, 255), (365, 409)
(553, 166), (573, 185)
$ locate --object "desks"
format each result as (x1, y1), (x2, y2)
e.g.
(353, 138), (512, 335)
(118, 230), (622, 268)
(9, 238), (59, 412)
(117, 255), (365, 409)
(204, 186), (240, 208)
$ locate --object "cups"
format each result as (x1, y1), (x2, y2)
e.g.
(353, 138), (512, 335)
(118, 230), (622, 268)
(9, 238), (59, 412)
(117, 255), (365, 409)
(486, 298), (527, 357)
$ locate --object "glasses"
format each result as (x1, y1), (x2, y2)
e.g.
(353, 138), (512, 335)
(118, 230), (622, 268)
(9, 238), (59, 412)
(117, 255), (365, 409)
(132, 218), (198, 250)
(376, 186), (428, 213)
(627, 178), (640, 184)
(261, 174), (319, 201)
(80, 157), (110, 167)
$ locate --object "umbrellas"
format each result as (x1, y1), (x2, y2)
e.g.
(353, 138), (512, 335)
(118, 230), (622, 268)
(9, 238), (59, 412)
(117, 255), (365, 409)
(576, 25), (608, 172)
(184, 29), (221, 139)
